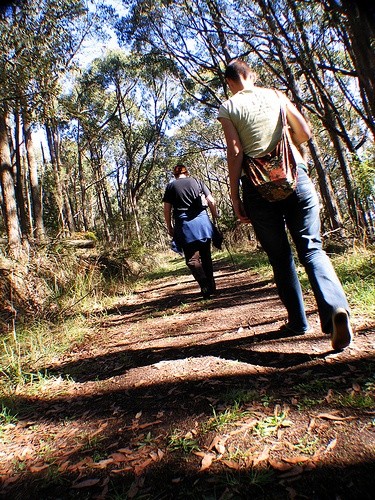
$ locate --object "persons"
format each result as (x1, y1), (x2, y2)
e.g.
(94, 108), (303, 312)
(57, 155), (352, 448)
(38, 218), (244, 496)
(217, 60), (353, 353)
(163, 164), (221, 299)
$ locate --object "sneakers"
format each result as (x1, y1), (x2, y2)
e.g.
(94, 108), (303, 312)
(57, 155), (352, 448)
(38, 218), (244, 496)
(329, 307), (352, 350)
(285, 320), (306, 334)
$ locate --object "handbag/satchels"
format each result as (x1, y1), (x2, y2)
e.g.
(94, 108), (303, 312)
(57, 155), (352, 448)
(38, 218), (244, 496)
(193, 175), (208, 208)
(242, 128), (296, 202)
(212, 219), (223, 251)
